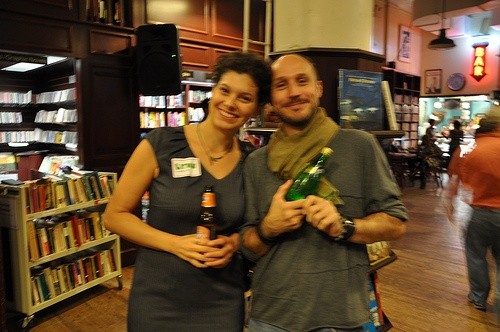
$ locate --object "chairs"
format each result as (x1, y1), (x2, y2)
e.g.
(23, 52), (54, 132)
(420, 152), (442, 190)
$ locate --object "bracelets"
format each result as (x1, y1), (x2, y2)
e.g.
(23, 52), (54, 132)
(255, 224), (278, 245)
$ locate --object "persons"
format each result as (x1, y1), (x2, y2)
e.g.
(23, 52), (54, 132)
(103, 52), (271, 332)
(418, 109), (500, 311)
(237, 55), (410, 332)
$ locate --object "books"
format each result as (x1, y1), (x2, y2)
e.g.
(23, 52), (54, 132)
(0, 90), (213, 306)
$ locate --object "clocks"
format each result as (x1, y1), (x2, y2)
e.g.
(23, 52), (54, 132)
(446, 73), (464, 91)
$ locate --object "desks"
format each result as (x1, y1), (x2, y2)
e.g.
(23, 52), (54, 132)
(388, 152), (416, 187)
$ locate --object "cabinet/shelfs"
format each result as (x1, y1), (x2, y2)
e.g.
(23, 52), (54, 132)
(381, 64), (422, 150)
(0, 169), (124, 332)
(88, 51), (218, 267)
(0, 75), (81, 181)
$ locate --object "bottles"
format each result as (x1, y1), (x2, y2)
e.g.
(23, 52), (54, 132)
(285, 147), (336, 202)
(196, 185), (218, 240)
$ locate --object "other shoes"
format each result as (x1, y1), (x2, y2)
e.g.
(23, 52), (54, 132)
(467, 294), (487, 309)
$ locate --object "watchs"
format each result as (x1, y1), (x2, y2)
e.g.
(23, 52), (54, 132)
(331, 216), (355, 244)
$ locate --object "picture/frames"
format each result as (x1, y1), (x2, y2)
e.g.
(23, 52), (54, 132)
(397, 23), (414, 64)
(424, 68), (442, 94)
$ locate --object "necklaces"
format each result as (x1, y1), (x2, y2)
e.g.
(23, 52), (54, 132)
(196, 121), (233, 165)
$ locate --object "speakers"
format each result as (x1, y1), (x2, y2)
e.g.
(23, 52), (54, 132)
(136, 23), (183, 96)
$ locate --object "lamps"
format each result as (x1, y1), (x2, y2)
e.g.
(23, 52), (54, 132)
(427, 0), (456, 48)
